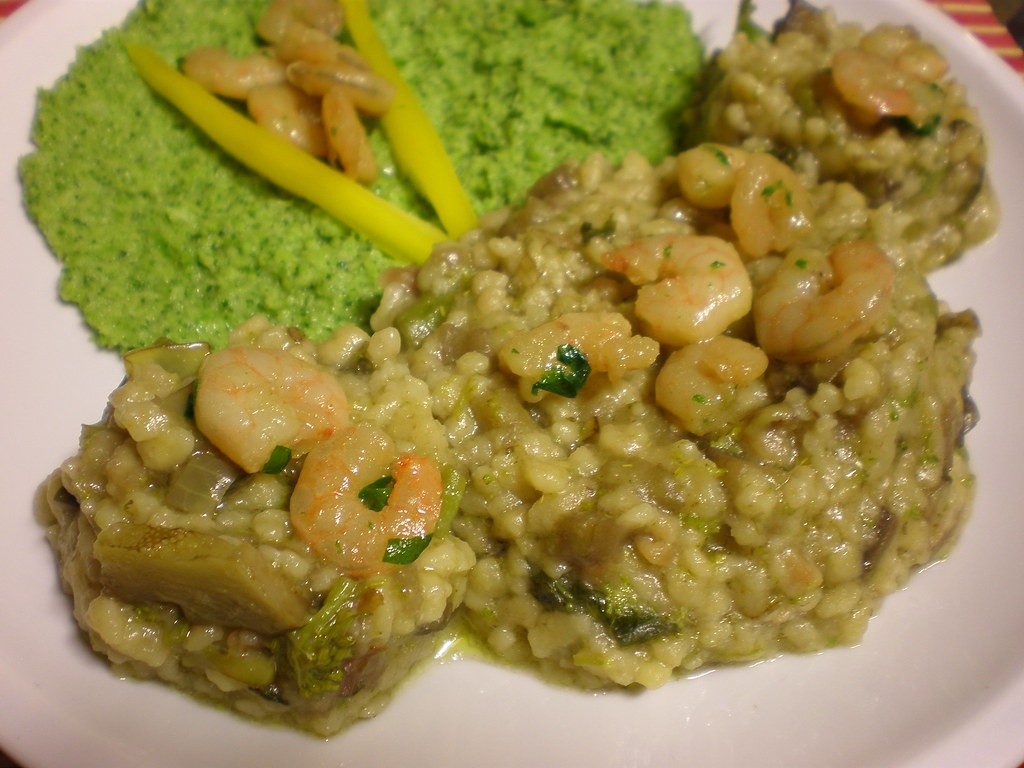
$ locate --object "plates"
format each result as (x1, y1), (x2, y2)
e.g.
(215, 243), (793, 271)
(0, 0), (1024, 768)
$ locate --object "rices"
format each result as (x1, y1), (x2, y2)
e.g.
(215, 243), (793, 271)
(44, 0), (1001, 741)
